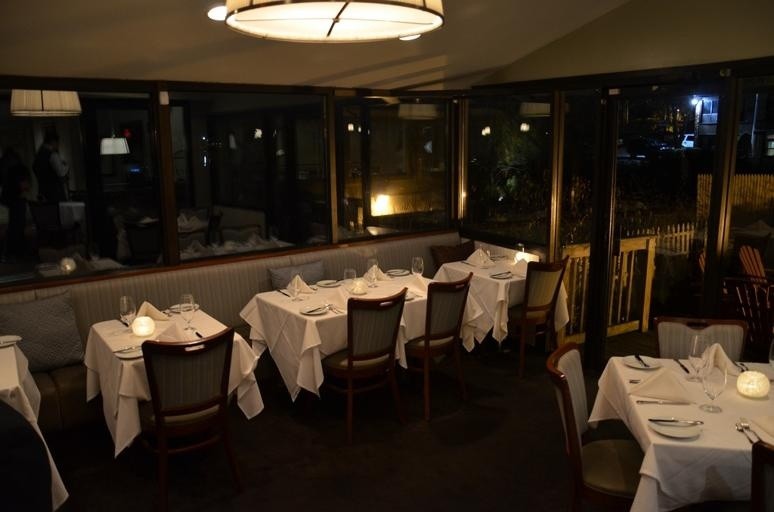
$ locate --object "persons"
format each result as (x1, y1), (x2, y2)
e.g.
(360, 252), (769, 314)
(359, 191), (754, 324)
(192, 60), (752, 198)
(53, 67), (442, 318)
(32, 133), (70, 208)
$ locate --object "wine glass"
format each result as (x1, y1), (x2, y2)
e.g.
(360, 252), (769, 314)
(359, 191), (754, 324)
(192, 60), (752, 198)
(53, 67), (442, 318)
(180, 294), (195, 330)
(366, 259), (378, 287)
(120, 296), (136, 333)
(699, 363), (726, 412)
(411, 256), (423, 278)
(344, 269), (356, 297)
(690, 333), (710, 381)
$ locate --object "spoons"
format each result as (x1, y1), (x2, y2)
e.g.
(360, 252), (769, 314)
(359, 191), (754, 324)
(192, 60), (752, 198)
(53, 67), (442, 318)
(735, 423), (753, 444)
(0, 341), (22, 345)
(648, 421), (704, 427)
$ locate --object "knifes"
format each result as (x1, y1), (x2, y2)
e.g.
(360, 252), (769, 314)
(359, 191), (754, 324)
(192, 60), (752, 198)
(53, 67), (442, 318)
(306, 308), (320, 313)
(673, 357), (689, 374)
(276, 288), (290, 297)
(190, 327), (202, 338)
(634, 354), (649, 367)
(115, 317), (128, 327)
(491, 271), (510, 276)
(623, 355), (661, 370)
(636, 399), (692, 406)
(112, 346), (141, 353)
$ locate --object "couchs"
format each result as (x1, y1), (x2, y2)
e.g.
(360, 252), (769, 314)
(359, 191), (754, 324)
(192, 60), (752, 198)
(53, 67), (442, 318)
(1, 226), (474, 430)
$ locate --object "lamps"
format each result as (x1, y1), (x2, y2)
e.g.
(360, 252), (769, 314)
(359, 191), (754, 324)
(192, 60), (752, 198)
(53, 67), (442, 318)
(520, 102), (551, 118)
(223, 0), (445, 47)
(397, 103), (441, 121)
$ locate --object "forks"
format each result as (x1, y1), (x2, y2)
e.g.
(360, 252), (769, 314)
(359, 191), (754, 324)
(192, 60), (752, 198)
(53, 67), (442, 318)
(740, 416), (763, 440)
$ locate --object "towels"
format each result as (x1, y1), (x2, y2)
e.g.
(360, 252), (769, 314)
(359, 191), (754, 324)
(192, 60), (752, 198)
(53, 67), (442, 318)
(696, 344), (741, 377)
(401, 273), (428, 298)
(134, 301), (170, 321)
(627, 369), (708, 404)
(155, 322), (192, 342)
(466, 249), (494, 267)
(362, 264), (386, 283)
(322, 287), (350, 311)
(508, 259), (528, 278)
(287, 274), (312, 297)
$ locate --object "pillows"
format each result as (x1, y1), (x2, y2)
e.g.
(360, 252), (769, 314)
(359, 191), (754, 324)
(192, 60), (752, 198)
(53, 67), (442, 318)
(265, 260), (330, 291)
(0, 288), (85, 375)
(430, 238), (475, 266)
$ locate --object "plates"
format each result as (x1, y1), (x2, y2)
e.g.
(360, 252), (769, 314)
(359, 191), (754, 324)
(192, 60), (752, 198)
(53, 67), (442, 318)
(299, 303), (328, 315)
(113, 345), (143, 360)
(316, 280), (340, 288)
(170, 303), (199, 314)
(0, 335), (22, 348)
(490, 271), (511, 278)
(387, 269), (410, 275)
(649, 414), (701, 437)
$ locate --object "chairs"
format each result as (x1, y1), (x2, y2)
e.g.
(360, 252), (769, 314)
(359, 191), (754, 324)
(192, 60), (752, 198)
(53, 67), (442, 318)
(545, 341), (643, 509)
(654, 314), (750, 361)
(321, 288), (409, 433)
(140, 326), (245, 510)
(508, 255), (570, 376)
(405, 273), (474, 421)
(721, 277), (773, 360)
